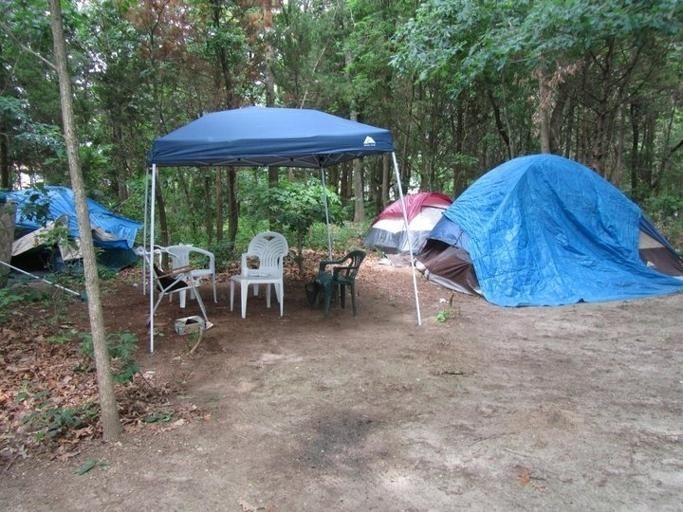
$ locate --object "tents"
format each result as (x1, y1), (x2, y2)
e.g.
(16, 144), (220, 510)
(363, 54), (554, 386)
(0, 185), (144, 278)
(414, 153), (683, 308)
(362, 191), (453, 256)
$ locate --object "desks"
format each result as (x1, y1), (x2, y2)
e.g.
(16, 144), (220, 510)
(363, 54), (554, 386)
(230, 275), (284, 318)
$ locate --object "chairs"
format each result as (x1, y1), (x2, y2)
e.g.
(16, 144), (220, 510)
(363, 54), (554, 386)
(312, 250), (366, 319)
(136, 244), (218, 327)
(229, 232), (289, 319)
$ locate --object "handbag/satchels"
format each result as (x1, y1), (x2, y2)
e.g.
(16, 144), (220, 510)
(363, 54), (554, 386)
(174, 316), (205, 336)
(154, 263), (188, 292)
